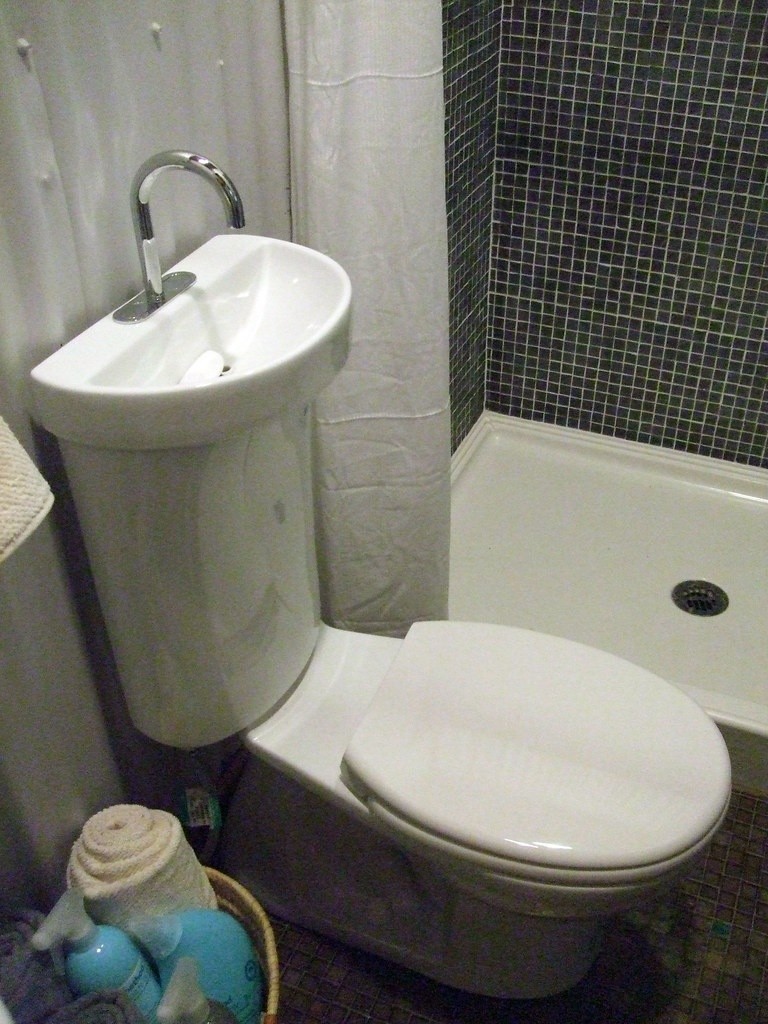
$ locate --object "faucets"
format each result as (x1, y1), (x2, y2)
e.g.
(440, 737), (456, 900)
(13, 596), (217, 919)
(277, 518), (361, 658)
(107, 149), (248, 324)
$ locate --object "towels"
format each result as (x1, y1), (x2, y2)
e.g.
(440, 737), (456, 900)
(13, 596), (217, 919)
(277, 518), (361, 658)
(0, 414), (55, 562)
(0, 894), (145, 1024)
(64, 801), (223, 934)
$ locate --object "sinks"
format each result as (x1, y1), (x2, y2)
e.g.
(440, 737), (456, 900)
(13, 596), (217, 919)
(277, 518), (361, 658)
(26, 232), (356, 456)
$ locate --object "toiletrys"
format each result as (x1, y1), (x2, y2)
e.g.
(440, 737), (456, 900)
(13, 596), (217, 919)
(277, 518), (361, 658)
(30, 885), (266, 1024)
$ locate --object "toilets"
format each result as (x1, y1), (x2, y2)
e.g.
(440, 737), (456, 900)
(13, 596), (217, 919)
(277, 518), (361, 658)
(50, 419), (733, 998)
